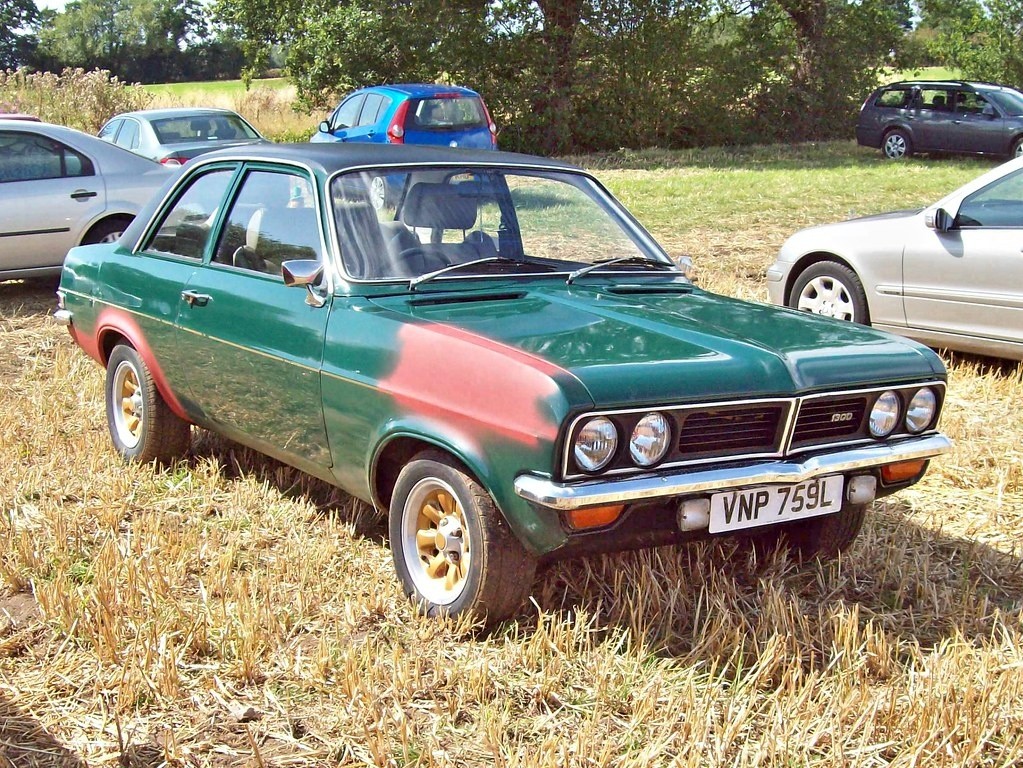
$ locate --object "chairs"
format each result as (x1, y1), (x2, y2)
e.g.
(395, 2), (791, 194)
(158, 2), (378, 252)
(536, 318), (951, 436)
(246, 204), (322, 271)
(190, 121), (212, 140)
(384, 182), (499, 274)
(957, 93), (968, 110)
(932, 95), (948, 110)
(428, 105), (443, 123)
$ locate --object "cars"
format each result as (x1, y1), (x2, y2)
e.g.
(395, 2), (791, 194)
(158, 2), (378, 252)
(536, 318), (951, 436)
(1, 119), (202, 282)
(52, 143), (952, 635)
(96, 107), (290, 207)
(766, 153), (1023, 360)
(307, 84), (502, 210)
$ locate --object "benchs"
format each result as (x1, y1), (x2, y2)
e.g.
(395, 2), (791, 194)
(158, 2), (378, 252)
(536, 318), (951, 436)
(166, 201), (427, 272)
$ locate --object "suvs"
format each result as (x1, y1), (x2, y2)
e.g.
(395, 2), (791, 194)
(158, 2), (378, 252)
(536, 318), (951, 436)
(855, 80), (1023, 161)
